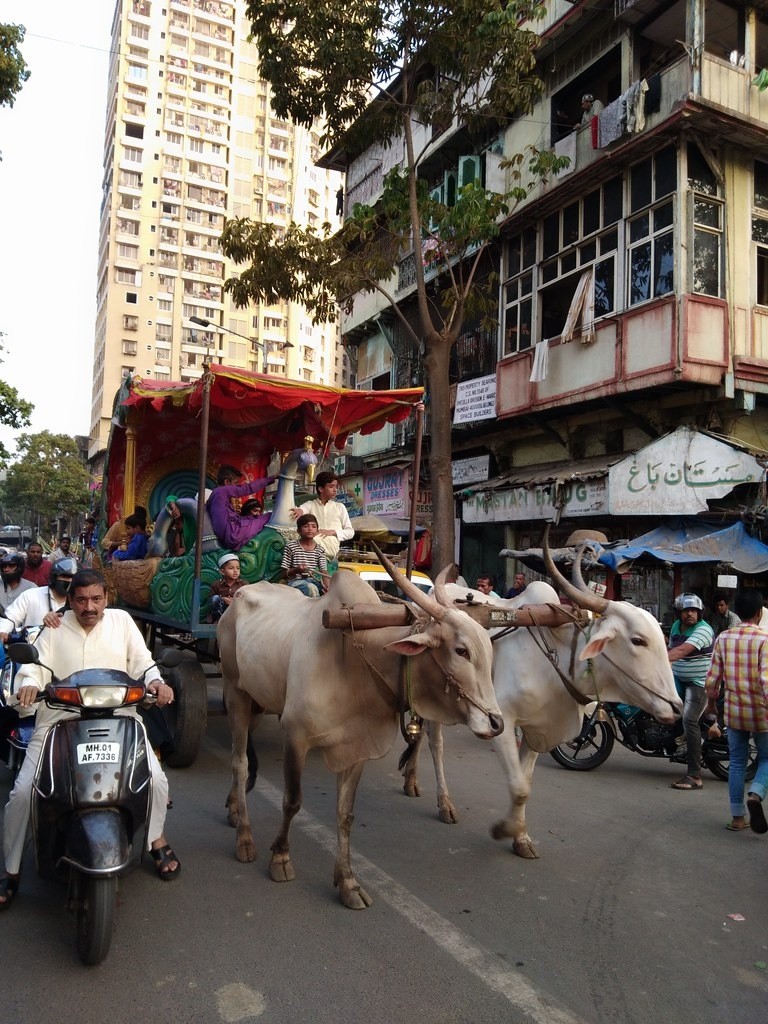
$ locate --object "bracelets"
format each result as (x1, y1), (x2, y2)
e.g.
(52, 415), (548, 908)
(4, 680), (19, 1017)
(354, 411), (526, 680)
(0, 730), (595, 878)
(287, 571), (294, 579)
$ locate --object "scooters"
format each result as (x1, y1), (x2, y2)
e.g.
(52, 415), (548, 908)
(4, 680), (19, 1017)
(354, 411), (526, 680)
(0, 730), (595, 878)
(6, 642), (197, 966)
(0, 603), (44, 780)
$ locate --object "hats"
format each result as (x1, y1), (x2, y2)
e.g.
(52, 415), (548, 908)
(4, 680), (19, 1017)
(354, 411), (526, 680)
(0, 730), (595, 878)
(218, 553), (240, 568)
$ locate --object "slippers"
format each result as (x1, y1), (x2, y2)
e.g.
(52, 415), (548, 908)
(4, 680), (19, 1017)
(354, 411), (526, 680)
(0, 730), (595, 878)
(746, 799), (768, 834)
(672, 777), (704, 790)
(726, 817), (750, 831)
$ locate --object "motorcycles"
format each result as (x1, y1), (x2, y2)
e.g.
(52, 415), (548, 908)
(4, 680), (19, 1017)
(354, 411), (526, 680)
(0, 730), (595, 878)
(550, 700), (761, 782)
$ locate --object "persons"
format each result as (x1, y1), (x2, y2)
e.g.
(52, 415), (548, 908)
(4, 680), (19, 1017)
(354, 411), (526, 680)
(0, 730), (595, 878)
(165, 495), (186, 558)
(581, 94), (605, 126)
(291, 472), (355, 563)
(476, 575), (501, 599)
(91, 510), (100, 548)
(206, 466), (280, 551)
(709, 595), (741, 638)
(281, 514), (330, 597)
(0, 537), (182, 911)
(100, 506), (146, 551)
(208, 554), (249, 624)
(241, 499), (261, 516)
(80, 518), (95, 549)
(507, 572), (526, 598)
(705, 589), (768, 833)
(667, 592), (721, 790)
(454, 564), (468, 588)
(112, 513), (151, 560)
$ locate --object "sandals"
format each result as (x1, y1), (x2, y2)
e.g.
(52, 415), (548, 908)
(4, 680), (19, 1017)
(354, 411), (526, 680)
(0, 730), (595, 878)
(149, 843), (181, 880)
(0, 871), (22, 908)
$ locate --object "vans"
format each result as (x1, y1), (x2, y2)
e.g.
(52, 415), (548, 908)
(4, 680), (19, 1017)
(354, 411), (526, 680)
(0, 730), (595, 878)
(2, 526), (20, 533)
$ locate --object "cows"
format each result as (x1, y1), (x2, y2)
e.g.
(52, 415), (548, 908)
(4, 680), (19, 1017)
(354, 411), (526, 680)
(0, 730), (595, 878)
(397, 524), (684, 859)
(214, 539), (504, 910)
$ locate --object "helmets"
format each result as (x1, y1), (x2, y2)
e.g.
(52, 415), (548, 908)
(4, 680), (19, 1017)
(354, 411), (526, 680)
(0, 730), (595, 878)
(47, 557), (83, 594)
(675, 592), (703, 622)
(0, 553), (26, 581)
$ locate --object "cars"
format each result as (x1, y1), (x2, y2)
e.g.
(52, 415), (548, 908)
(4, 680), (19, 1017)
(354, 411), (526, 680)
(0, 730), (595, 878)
(337, 562), (437, 603)
